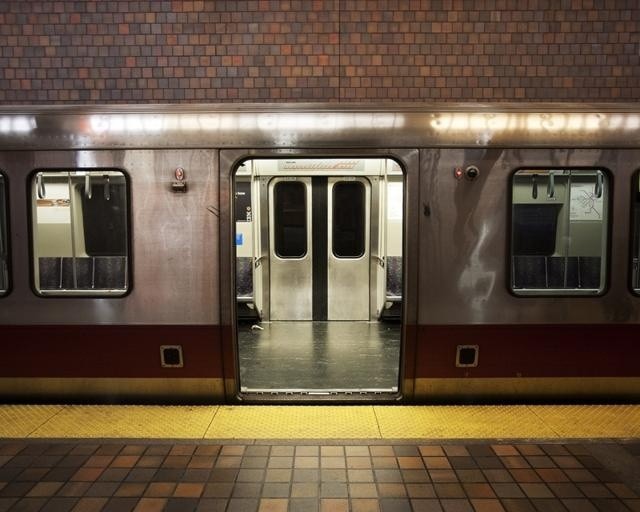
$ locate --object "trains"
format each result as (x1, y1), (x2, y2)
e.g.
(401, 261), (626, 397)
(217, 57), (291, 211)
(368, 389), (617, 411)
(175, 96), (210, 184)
(0, 104), (640, 401)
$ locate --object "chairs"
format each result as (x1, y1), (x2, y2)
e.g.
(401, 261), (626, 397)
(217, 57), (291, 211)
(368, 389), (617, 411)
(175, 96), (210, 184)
(38, 255), (263, 324)
(377, 257), (640, 321)
